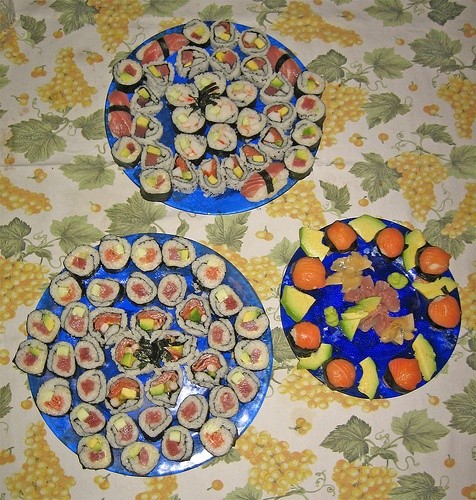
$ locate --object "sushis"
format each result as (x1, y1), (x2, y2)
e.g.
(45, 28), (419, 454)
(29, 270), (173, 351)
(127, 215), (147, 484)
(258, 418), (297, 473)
(12, 234), (271, 477)
(279, 214), (463, 400)
(107, 18), (327, 203)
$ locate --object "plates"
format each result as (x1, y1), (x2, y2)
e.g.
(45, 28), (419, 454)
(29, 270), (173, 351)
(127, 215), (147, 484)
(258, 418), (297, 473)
(280, 217), (461, 400)
(103, 21), (321, 216)
(25, 232), (272, 478)
